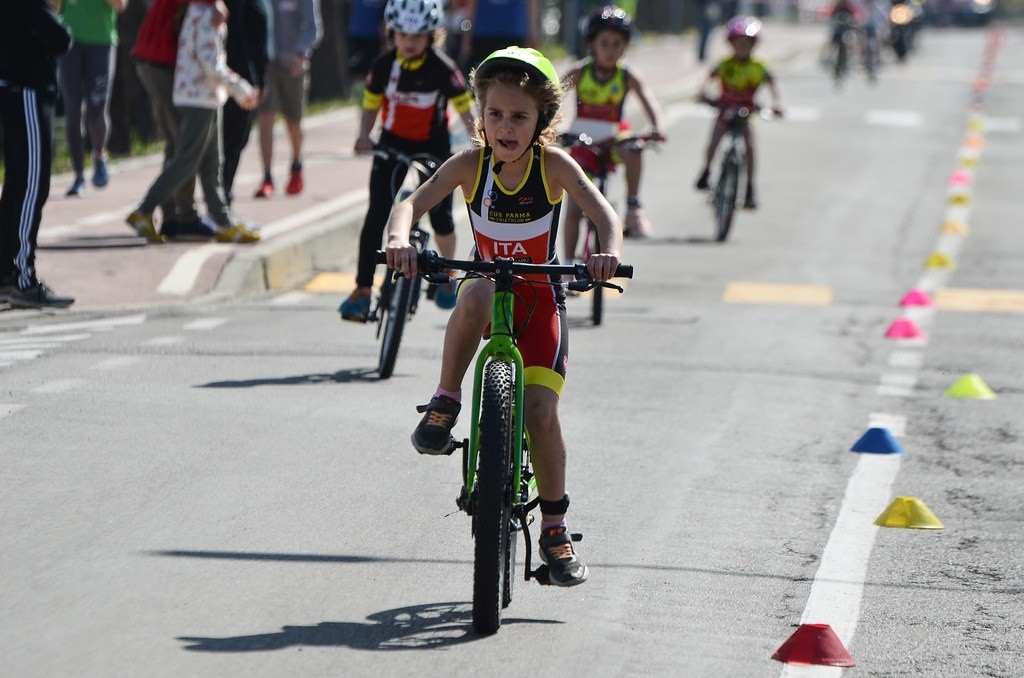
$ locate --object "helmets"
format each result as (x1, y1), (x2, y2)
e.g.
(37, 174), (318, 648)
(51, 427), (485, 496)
(728, 17), (761, 42)
(383, 0), (439, 35)
(581, 8), (631, 44)
(474, 45), (562, 120)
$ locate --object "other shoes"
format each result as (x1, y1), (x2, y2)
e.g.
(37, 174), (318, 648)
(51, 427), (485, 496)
(68, 178), (86, 194)
(94, 157), (109, 187)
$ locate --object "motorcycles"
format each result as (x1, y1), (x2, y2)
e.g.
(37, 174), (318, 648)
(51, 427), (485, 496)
(890, 2), (917, 62)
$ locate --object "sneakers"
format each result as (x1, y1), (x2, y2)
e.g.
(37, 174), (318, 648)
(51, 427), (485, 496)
(340, 288), (371, 321)
(436, 269), (459, 310)
(159, 215), (215, 241)
(539, 524), (587, 586)
(215, 224), (260, 241)
(128, 213), (165, 243)
(287, 167), (302, 195)
(411, 394), (463, 455)
(10, 281), (75, 309)
(256, 182), (272, 197)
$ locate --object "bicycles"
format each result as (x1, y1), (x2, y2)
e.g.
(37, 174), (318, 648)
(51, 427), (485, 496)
(353, 143), (454, 379)
(697, 93), (784, 243)
(819, 15), (885, 87)
(555, 131), (665, 328)
(369, 247), (638, 634)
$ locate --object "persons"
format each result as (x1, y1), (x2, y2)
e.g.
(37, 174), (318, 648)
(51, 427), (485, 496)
(388, 45), (621, 586)
(126, 0), (324, 244)
(51, 0), (129, 194)
(696, 15), (781, 208)
(831, 1), (922, 79)
(341, 0), (475, 323)
(0, 0), (74, 308)
(556, 8), (660, 293)
(445, 0), (473, 78)
